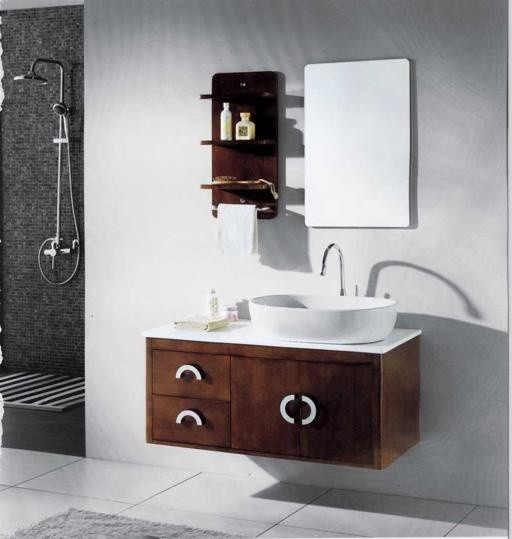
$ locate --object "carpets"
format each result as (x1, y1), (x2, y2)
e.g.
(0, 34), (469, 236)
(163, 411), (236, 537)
(3, 507), (250, 539)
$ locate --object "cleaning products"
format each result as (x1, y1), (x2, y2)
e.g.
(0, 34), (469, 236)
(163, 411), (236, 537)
(236, 112), (256, 139)
(220, 102), (231, 140)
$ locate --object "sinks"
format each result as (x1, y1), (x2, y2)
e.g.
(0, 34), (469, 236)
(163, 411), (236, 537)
(250, 294), (397, 342)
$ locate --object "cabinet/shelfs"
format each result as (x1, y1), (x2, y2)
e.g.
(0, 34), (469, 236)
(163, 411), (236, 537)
(146, 314), (422, 470)
(200, 70), (279, 219)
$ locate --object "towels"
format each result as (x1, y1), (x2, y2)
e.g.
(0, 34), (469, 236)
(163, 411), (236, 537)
(215, 202), (259, 256)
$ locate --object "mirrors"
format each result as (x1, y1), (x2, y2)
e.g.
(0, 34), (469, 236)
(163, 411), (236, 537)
(304, 58), (410, 228)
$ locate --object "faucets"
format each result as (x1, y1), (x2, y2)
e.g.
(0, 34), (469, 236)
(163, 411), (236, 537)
(320, 243), (346, 296)
